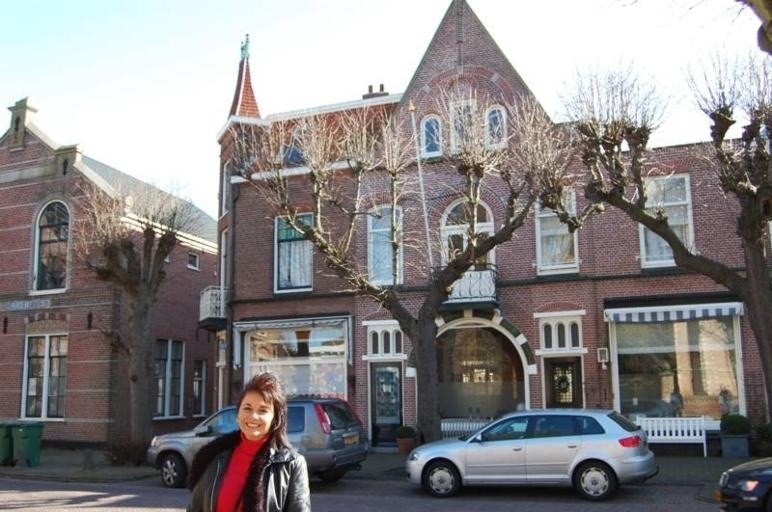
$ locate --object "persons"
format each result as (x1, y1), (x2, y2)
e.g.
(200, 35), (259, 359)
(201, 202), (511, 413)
(719, 384), (733, 415)
(670, 385), (683, 417)
(185, 370), (311, 512)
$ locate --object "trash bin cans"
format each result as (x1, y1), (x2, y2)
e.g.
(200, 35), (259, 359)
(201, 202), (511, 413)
(0, 420), (45, 467)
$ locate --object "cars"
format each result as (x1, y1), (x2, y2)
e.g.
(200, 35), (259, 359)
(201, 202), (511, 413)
(404, 405), (660, 502)
(619, 398), (676, 419)
(715, 457), (772, 512)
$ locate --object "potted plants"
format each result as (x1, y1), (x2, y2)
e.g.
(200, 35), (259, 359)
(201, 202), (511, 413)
(720, 413), (752, 458)
(395, 425), (416, 460)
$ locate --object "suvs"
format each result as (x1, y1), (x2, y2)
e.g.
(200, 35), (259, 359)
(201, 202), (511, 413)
(144, 397), (368, 491)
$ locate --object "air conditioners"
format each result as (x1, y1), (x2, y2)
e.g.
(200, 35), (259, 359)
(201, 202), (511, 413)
(199, 285), (224, 322)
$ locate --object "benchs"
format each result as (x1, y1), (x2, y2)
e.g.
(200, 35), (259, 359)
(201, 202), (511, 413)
(635, 414), (708, 459)
